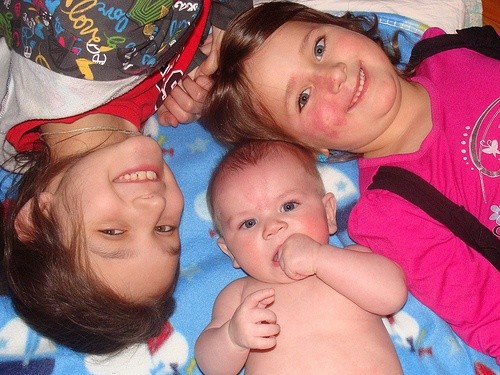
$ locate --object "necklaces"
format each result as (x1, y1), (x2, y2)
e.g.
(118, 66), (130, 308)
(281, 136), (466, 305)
(39, 127), (142, 141)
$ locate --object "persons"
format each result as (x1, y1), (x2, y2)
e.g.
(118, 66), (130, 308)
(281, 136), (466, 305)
(198, 1), (500, 362)
(194, 138), (408, 375)
(0, 0), (253, 354)
